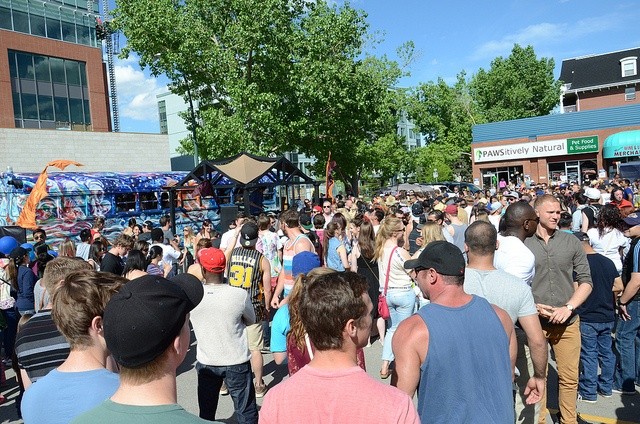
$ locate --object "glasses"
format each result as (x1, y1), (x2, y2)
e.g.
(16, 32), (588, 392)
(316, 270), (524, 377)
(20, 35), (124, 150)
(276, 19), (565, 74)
(323, 206), (330, 208)
(522, 217), (540, 225)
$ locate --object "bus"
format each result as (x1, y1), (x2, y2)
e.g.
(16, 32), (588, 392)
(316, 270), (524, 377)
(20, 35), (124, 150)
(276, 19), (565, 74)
(0, 167), (276, 253)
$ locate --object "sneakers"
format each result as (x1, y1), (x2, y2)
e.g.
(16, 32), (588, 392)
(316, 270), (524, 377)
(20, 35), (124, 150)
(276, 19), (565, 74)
(576, 393), (597, 403)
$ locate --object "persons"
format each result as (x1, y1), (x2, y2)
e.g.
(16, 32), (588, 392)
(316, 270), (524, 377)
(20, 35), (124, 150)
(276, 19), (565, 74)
(145, 247), (163, 275)
(332, 212), (350, 253)
(194, 220), (211, 248)
(88, 242), (105, 272)
(493, 178), (535, 203)
(91, 216), (102, 242)
(322, 199), (333, 227)
(371, 216), (415, 378)
(573, 233), (621, 380)
(413, 187), (494, 211)
(188, 247), (257, 424)
(16, 251), (39, 316)
(180, 225), (195, 264)
(616, 211), (640, 380)
(445, 203), (469, 267)
(493, 200), (536, 285)
(121, 217), (136, 236)
(101, 234), (134, 273)
(225, 217), (264, 281)
(220, 222), (272, 398)
(303, 200), (313, 211)
(227, 220), (238, 230)
(332, 195), (369, 223)
(159, 217), (181, 246)
(194, 238), (213, 252)
(219, 211), (248, 278)
(58, 241), (75, 257)
(15, 257), (94, 378)
(19, 274), (120, 423)
(255, 216), (282, 294)
(346, 214), (362, 238)
(139, 220), (153, 242)
(75, 229), (92, 260)
(369, 191), (413, 221)
(95, 235), (113, 249)
(148, 229), (183, 270)
(33, 228), (46, 242)
(350, 221), (380, 343)
(74, 273), (225, 424)
(34, 254), (52, 314)
(324, 213), (349, 272)
(122, 248), (148, 280)
(132, 224), (141, 238)
(314, 212), (325, 246)
(268, 213), (277, 228)
(462, 221), (548, 389)
(391, 240), (517, 424)
(411, 222), (447, 309)
(270, 211), (320, 365)
(312, 203), (322, 217)
(300, 211), (312, 230)
(425, 211), (456, 245)
(586, 203), (631, 276)
(1, 257), (18, 352)
(259, 273), (422, 424)
(269, 250), (320, 365)
(33, 242), (52, 254)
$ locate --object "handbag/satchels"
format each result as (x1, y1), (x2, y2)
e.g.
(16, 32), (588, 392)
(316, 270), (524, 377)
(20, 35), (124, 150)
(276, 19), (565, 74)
(378, 294), (398, 320)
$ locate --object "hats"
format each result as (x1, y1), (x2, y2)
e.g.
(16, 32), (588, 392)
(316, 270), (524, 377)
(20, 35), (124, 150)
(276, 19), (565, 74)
(313, 205), (322, 213)
(404, 240), (465, 276)
(300, 215), (313, 229)
(197, 247), (226, 272)
(240, 222), (259, 247)
(292, 251), (321, 279)
(583, 187), (600, 199)
(103, 273), (204, 369)
(622, 212), (640, 225)
